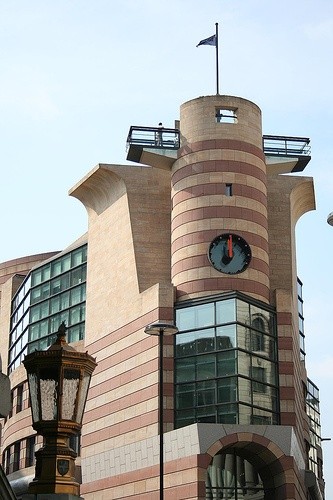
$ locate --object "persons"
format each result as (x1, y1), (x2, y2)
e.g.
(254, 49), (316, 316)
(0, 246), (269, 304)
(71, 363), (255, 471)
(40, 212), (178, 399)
(156, 122), (165, 146)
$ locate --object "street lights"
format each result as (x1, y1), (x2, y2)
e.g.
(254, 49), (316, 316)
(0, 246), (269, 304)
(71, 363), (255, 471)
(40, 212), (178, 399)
(143, 323), (178, 500)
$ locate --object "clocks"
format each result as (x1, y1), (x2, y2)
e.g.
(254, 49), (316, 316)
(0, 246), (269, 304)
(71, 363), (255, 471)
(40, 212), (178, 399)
(207, 233), (251, 275)
